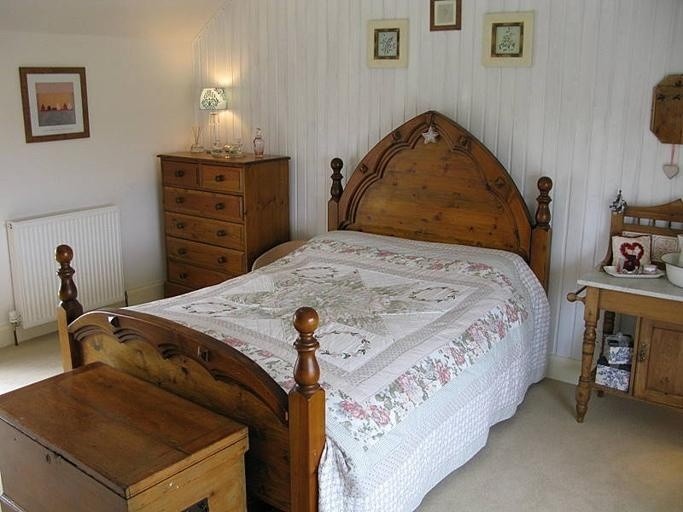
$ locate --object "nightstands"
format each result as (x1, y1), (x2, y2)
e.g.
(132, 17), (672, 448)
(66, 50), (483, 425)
(567, 196), (683, 423)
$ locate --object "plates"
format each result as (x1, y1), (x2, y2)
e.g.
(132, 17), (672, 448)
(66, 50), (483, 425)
(603, 265), (664, 279)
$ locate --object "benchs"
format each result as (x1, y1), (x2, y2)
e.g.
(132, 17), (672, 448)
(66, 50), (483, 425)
(0, 361), (251, 512)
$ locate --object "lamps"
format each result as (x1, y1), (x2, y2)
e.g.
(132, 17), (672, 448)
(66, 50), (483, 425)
(199, 87), (228, 154)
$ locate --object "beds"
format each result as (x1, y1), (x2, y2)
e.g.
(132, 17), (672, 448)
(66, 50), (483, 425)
(55, 109), (553, 511)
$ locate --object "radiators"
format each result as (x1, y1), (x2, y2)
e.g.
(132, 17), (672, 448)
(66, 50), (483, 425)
(5, 202), (126, 330)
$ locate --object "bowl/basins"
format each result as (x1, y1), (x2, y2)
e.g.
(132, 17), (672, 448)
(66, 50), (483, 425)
(661, 251), (683, 287)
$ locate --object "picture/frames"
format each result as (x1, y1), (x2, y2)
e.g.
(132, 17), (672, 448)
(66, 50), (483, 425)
(481, 11), (534, 66)
(369, 19), (409, 67)
(429, 0), (461, 31)
(19, 67), (90, 144)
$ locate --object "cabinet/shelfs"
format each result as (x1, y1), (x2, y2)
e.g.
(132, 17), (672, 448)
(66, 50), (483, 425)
(158, 151), (291, 298)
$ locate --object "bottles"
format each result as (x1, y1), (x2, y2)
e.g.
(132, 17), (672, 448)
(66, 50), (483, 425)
(254, 128), (264, 158)
